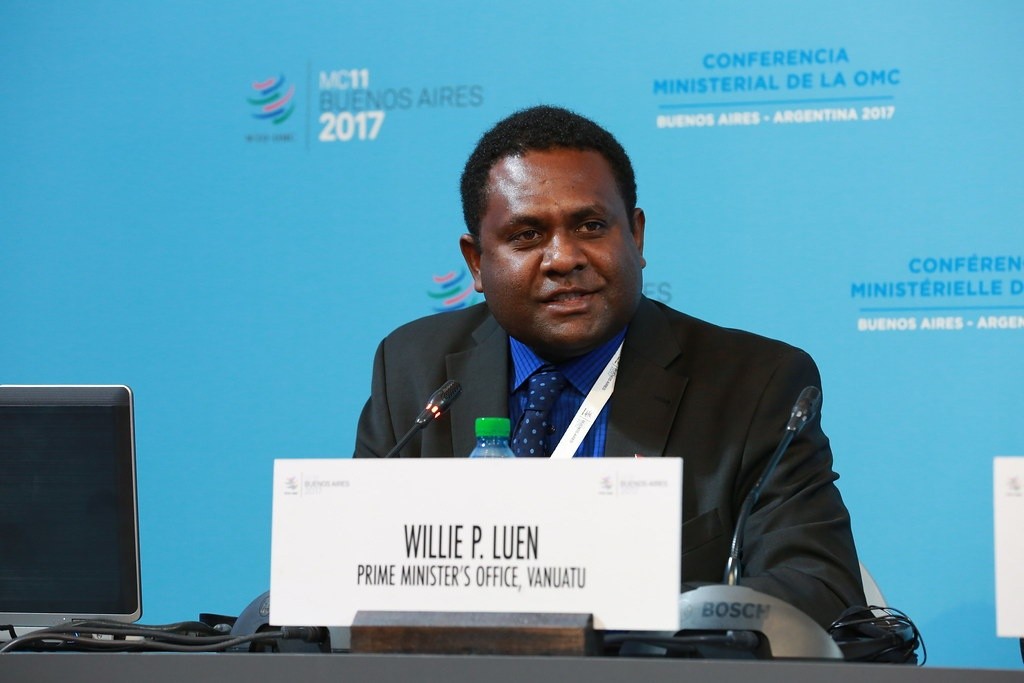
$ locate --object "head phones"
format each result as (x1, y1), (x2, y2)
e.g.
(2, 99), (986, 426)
(824, 606), (922, 665)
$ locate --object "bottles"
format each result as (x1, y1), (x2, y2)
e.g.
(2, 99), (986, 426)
(470, 416), (515, 457)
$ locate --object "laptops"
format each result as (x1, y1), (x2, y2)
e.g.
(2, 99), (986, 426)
(0, 384), (159, 646)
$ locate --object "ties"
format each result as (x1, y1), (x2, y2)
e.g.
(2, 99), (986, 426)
(512, 364), (568, 458)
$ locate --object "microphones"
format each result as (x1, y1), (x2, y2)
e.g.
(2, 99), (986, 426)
(232, 380), (465, 655)
(681, 387), (846, 662)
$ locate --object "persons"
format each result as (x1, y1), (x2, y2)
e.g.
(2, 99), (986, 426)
(351, 105), (869, 637)
